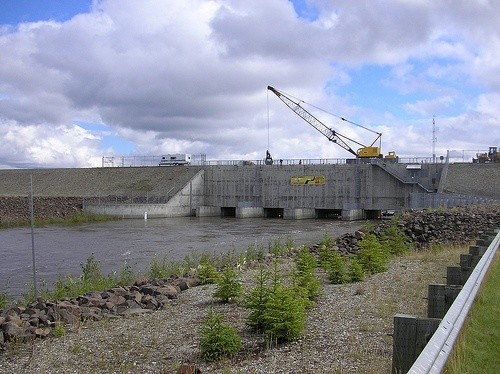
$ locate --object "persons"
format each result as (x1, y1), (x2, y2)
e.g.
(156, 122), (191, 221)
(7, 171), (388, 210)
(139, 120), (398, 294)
(280, 158), (301, 166)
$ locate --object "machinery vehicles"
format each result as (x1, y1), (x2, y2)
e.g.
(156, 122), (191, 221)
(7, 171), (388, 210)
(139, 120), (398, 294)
(473, 146), (500, 164)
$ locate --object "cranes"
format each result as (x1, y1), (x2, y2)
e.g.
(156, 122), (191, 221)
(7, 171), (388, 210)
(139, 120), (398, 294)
(265, 84), (382, 165)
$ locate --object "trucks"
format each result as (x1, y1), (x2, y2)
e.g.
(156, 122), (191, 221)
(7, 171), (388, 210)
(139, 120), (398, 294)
(159, 153), (191, 166)
